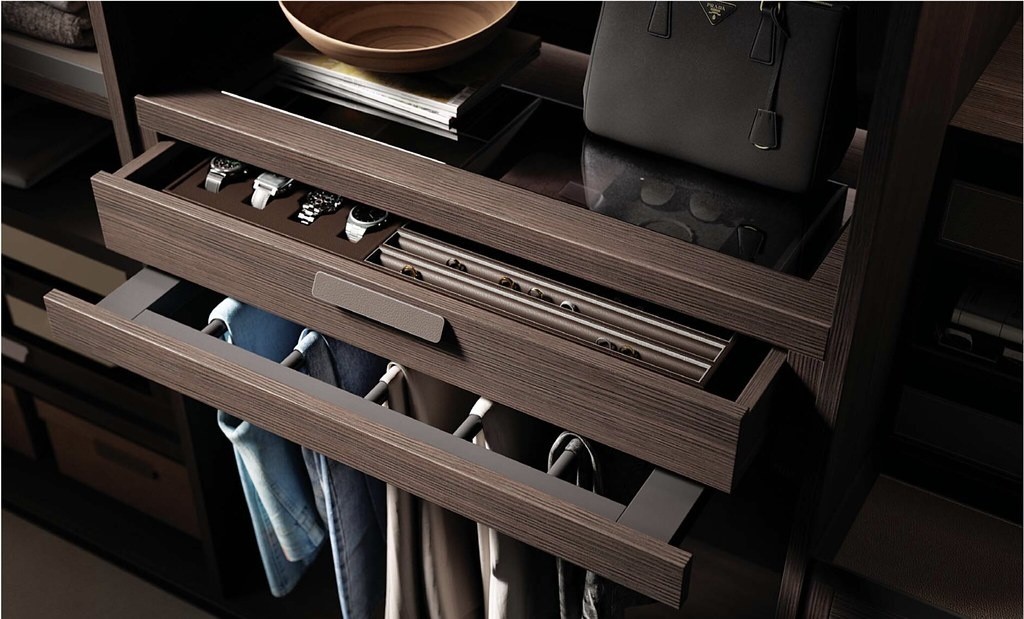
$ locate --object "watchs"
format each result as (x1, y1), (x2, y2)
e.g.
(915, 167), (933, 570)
(251, 171), (295, 209)
(346, 204), (388, 242)
(205, 155), (247, 193)
(298, 189), (342, 225)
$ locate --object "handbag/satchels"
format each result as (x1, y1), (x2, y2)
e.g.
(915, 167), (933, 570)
(583, 1), (848, 192)
(581, 138), (801, 273)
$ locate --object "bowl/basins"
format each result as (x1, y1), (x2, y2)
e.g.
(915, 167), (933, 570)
(278, 1), (519, 73)
